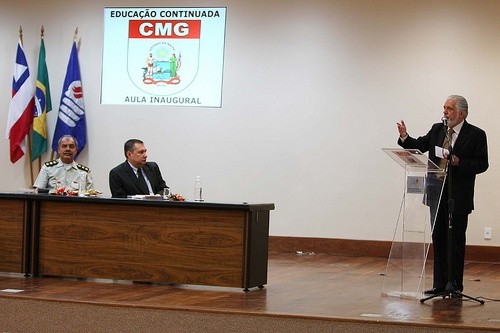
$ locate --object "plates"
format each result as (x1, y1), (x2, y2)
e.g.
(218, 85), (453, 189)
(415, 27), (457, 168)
(72, 190), (103, 196)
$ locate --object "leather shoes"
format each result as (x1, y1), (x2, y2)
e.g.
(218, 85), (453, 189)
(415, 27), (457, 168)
(424, 287), (443, 296)
(443, 290), (462, 299)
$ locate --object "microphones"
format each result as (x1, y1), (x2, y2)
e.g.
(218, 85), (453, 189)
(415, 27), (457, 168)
(441, 117), (447, 126)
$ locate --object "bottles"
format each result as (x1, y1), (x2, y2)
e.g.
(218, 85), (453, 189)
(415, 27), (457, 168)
(195, 176), (202, 200)
(78, 172), (86, 197)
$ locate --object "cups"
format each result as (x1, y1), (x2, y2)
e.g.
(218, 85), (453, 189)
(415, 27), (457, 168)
(163, 188), (170, 200)
(56, 183), (63, 196)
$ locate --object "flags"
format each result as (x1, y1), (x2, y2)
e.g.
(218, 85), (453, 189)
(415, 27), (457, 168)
(6, 37), (35, 163)
(32, 38), (52, 162)
(52, 42), (86, 160)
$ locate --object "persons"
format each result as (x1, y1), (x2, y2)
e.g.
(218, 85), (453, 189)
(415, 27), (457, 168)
(109, 139), (184, 286)
(397, 95), (489, 296)
(33, 135), (94, 280)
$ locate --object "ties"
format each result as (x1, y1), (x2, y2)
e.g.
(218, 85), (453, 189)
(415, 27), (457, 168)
(435, 129), (456, 178)
(136, 169), (150, 196)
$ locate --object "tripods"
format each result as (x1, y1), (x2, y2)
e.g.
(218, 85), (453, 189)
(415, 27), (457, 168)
(419, 127), (485, 305)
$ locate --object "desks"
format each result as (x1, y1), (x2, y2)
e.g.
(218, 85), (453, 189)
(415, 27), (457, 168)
(31, 194), (276, 292)
(0, 191), (32, 276)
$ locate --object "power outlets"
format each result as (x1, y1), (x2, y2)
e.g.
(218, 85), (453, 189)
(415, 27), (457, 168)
(484, 226), (492, 239)
